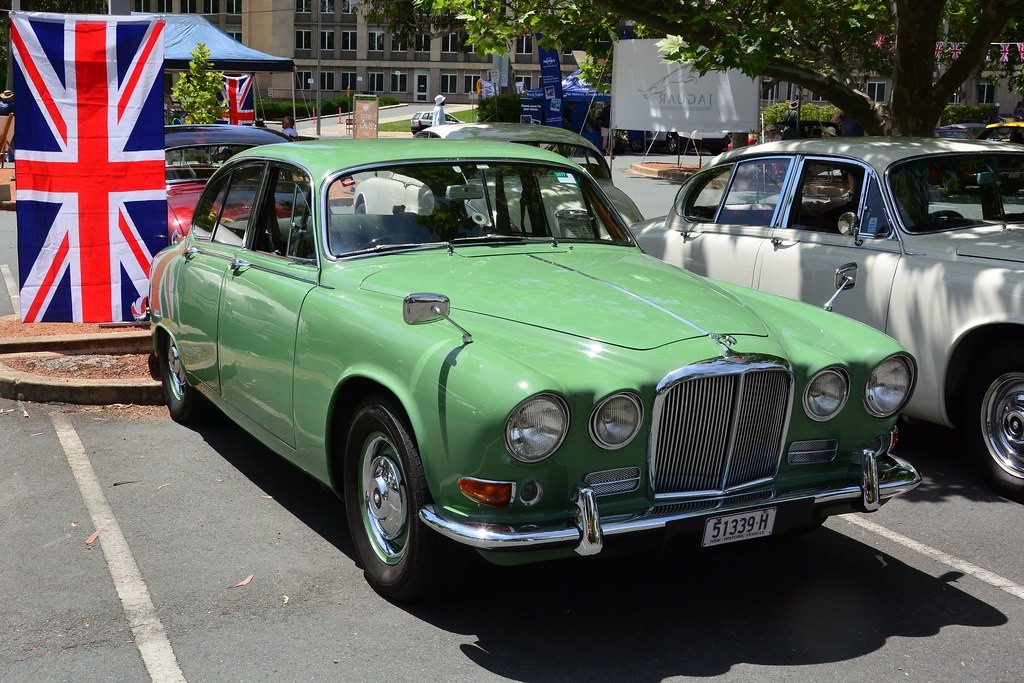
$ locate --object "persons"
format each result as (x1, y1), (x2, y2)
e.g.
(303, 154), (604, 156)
(753, 100), (866, 202)
(432, 95), (446, 126)
(0, 90), (15, 162)
(281, 116), (298, 137)
(994, 101), (1024, 139)
(596, 101), (615, 155)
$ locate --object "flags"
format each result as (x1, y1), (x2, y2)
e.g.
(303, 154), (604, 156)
(212, 73), (255, 153)
(889, 41), (896, 56)
(8, 9), (169, 323)
(984, 49), (991, 61)
(1017, 42), (1024, 63)
(872, 33), (887, 51)
(950, 42), (960, 60)
(935, 41), (943, 61)
(1000, 43), (1009, 62)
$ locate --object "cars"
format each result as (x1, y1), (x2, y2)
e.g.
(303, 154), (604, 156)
(352, 122), (645, 239)
(757, 120), (842, 181)
(927, 120), (1024, 191)
(630, 131), (730, 157)
(147, 135), (924, 600)
(628, 134), (1024, 500)
(165, 124), (311, 246)
(411, 111), (462, 133)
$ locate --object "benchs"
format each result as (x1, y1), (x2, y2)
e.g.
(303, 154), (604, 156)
(226, 213), (480, 260)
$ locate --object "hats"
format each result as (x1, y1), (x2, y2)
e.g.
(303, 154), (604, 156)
(435, 94), (446, 106)
(825, 126), (839, 136)
(764, 123), (776, 131)
(788, 100), (798, 110)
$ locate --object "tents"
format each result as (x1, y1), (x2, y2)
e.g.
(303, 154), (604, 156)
(520, 69), (643, 155)
(111, 12), (295, 135)
